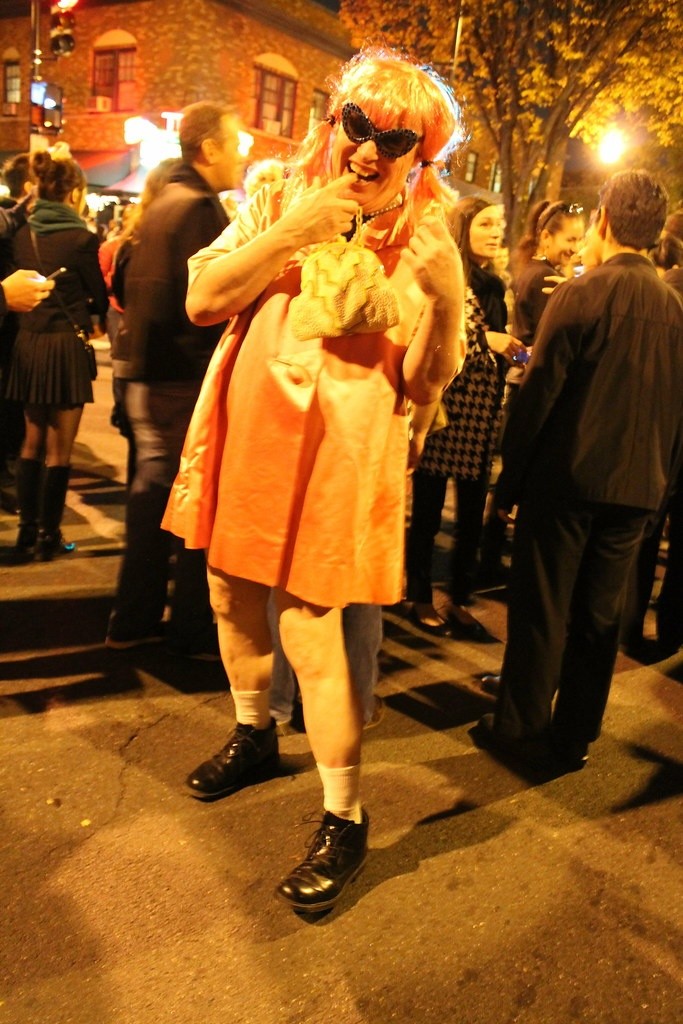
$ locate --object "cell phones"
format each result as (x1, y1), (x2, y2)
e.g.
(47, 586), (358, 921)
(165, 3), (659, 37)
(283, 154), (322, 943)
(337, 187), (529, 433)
(45, 267), (67, 281)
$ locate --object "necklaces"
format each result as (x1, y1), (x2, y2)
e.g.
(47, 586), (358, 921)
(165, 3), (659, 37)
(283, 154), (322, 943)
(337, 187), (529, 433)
(362, 200), (403, 217)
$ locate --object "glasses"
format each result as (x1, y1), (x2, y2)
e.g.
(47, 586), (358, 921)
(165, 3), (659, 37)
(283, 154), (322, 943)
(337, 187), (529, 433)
(542, 201), (583, 228)
(341, 103), (426, 160)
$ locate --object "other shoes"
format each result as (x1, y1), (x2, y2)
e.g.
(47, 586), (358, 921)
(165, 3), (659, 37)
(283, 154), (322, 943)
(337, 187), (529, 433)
(0, 490), (20, 515)
(269, 706), (293, 724)
(479, 713), (546, 784)
(0, 462), (16, 487)
(361, 695), (385, 729)
(472, 573), (508, 593)
(619, 637), (645, 656)
(104, 621), (170, 650)
(559, 739), (590, 772)
(481, 674), (501, 694)
(410, 605), (459, 640)
(445, 611), (488, 639)
(170, 623), (220, 661)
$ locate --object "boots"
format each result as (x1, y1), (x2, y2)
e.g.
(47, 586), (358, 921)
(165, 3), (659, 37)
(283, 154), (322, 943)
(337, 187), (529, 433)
(14, 460), (43, 561)
(33, 464), (75, 563)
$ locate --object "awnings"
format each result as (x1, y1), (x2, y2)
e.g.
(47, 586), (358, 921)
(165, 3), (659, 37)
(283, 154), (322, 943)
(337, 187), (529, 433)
(100, 165), (147, 197)
(69, 148), (131, 186)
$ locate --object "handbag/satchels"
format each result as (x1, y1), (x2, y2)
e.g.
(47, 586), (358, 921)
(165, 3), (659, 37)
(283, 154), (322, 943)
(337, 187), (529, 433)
(83, 342), (99, 383)
(287, 207), (400, 341)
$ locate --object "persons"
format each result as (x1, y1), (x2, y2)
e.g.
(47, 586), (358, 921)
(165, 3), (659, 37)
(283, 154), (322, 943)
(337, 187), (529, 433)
(0, 150), (249, 560)
(410, 200), (682, 637)
(158, 29), (468, 910)
(480, 167), (682, 769)
(97, 97), (250, 691)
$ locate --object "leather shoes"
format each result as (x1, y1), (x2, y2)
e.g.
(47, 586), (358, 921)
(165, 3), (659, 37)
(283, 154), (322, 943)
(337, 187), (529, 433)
(274, 807), (369, 912)
(183, 716), (281, 799)
